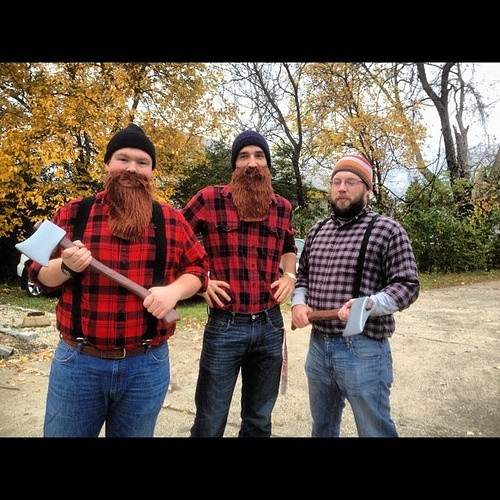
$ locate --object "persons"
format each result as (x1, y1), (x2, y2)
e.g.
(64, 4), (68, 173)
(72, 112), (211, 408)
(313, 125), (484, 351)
(181, 131), (299, 438)
(289, 155), (421, 438)
(20, 126), (210, 438)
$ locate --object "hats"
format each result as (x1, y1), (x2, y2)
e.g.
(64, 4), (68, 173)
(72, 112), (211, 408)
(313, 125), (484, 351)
(230, 130), (271, 169)
(104, 124), (155, 171)
(331, 154), (372, 189)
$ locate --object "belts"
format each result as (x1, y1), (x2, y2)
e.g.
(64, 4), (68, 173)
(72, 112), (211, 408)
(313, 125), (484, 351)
(61, 335), (169, 359)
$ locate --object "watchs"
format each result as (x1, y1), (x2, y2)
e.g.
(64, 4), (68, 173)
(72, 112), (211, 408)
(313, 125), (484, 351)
(281, 272), (297, 284)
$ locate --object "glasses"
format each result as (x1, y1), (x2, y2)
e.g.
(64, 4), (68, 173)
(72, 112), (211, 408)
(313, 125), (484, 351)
(328, 180), (364, 187)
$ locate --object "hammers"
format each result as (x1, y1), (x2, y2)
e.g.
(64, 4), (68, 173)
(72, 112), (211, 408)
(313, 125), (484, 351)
(15, 219), (179, 328)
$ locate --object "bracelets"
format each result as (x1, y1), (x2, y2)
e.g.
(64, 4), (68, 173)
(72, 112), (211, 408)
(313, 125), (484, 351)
(60, 261), (81, 276)
